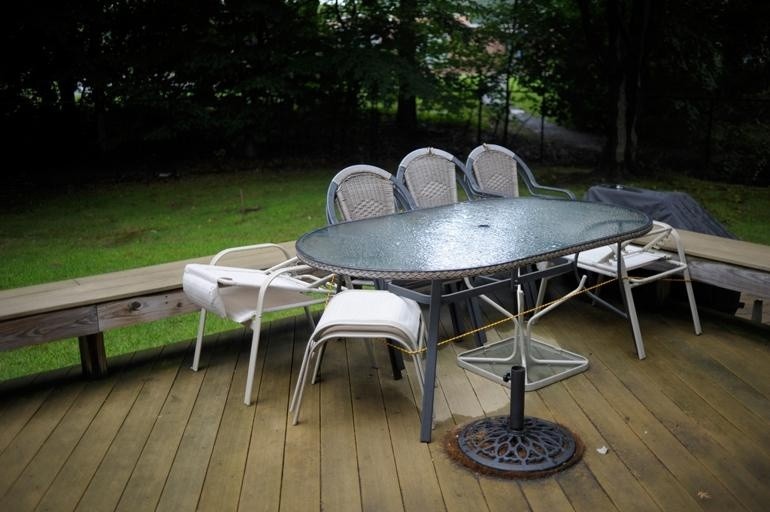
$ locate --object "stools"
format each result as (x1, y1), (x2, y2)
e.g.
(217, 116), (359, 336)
(291, 335), (437, 427)
(290, 288), (429, 410)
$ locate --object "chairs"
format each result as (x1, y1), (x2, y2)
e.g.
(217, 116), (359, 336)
(327, 161), (493, 368)
(402, 176), (534, 348)
(465, 143), (577, 309)
(576, 264), (577, 300)
(181, 243), (378, 406)
(396, 148), (539, 343)
(465, 169), (566, 316)
(535, 217), (704, 361)
(326, 188), (493, 380)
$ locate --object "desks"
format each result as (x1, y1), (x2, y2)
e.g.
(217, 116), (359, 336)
(298, 198), (653, 443)
